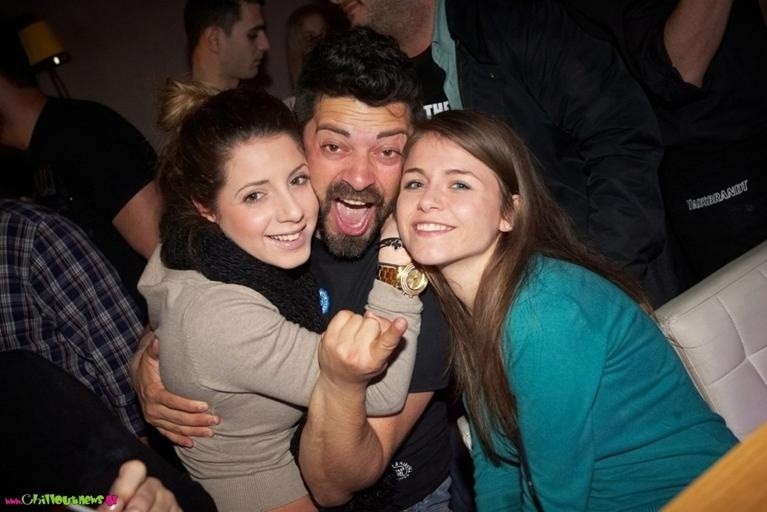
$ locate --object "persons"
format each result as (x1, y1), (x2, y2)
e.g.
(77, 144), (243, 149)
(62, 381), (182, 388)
(1, 0), (765, 511)
